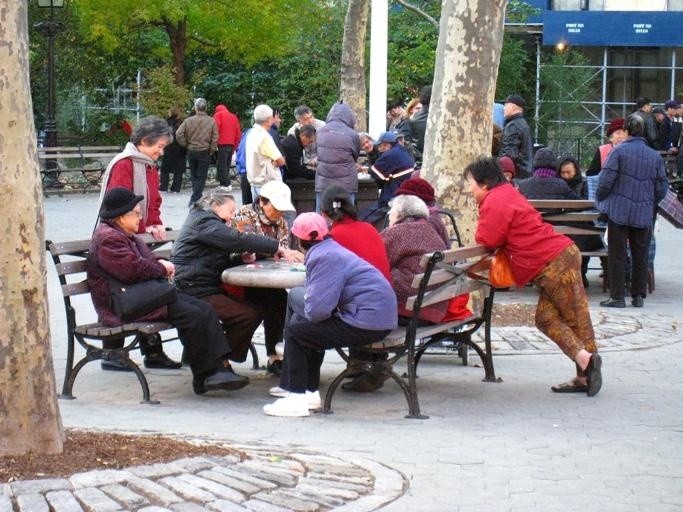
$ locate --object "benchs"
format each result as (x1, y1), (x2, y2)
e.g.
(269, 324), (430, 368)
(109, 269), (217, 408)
(656, 149), (683, 200)
(177, 155), (239, 186)
(519, 198), (616, 294)
(316, 245), (501, 418)
(44, 230), (260, 404)
(28, 140), (125, 193)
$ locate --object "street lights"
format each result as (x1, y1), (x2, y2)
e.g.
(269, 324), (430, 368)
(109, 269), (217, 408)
(33, 1), (64, 192)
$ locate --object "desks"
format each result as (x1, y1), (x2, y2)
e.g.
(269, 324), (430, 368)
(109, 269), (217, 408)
(226, 251), (315, 289)
(289, 174), (379, 220)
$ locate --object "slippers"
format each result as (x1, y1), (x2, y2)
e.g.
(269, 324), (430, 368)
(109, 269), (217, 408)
(577, 354), (602, 396)
(551, 378), (588, 392)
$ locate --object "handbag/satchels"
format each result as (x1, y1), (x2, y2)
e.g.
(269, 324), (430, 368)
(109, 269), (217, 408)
(108, 279), (177, 321)
(488, 249), (515, 288)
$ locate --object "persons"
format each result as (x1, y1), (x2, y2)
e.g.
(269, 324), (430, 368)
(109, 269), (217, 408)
(263, 211), (399, 417)
(101, 86), (683, 394)
(86, 185), (251, 394)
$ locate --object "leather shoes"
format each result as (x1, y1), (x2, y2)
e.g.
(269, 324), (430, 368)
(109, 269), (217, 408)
(632, 295), (644, 307)
(101, 342), (385, 417)
(600, 298), (625, 307)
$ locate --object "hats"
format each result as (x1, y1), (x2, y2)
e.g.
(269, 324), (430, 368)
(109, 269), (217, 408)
(290, 212), (329, 241)
(100, 188), (144, 218)
(260, 180), (297, 212)
